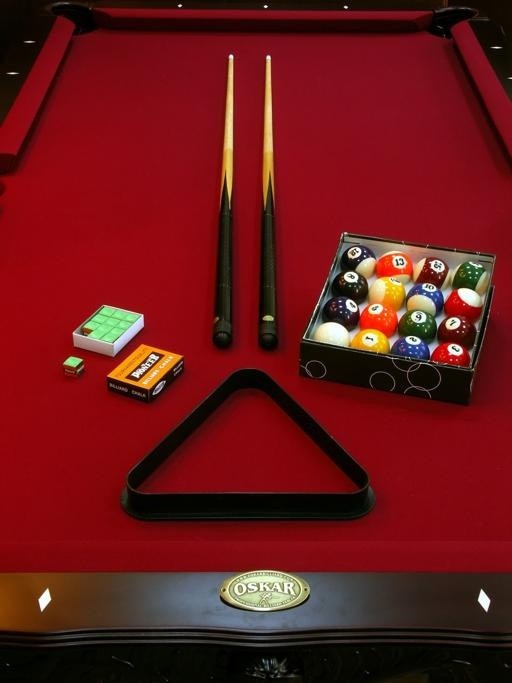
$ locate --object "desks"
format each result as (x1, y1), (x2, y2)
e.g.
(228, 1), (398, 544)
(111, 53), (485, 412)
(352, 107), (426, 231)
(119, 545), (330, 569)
(0, 1), (512, 683)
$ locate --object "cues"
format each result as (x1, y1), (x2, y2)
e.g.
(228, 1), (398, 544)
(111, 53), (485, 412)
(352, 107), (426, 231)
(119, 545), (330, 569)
(259, 56), (277, 348)
(211, 53), (234, 347)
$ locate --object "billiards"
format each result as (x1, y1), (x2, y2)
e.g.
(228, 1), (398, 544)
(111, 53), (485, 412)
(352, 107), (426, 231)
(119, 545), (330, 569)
(315, 245), (491, 368)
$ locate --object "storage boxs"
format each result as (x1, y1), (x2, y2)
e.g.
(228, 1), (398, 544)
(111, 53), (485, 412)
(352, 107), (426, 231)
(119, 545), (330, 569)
(297, 230), (497, 407)
